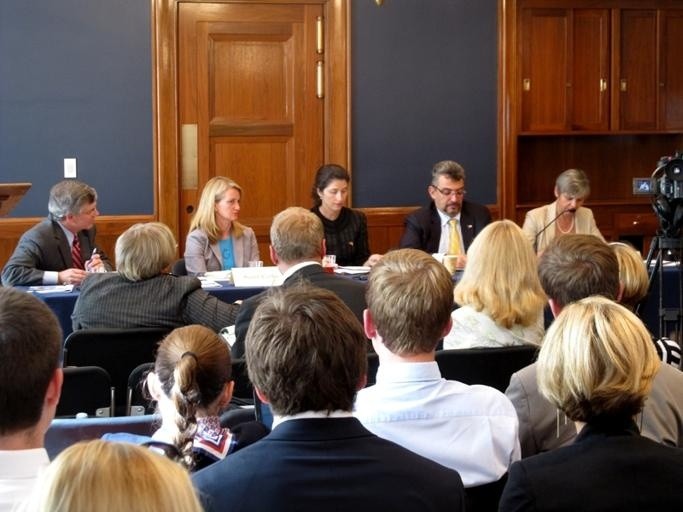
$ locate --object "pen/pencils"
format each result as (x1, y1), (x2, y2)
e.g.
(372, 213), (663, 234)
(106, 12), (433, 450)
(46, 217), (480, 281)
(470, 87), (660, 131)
(86, 248), (97, 271)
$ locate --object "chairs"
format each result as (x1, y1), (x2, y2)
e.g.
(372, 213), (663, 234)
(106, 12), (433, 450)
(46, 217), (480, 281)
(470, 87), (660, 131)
(56, 326), (177, 371)
(169, 259), (190, 280)
(122, 360), (260, 420)
(55, 367), (122, 420)
(433, 345), (540, 393)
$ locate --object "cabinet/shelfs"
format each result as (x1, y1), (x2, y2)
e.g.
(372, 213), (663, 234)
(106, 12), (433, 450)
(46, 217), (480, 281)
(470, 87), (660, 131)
(516, 1), (572, 132)
(571, 2), (661, 133)
(659, 0), (681, 134)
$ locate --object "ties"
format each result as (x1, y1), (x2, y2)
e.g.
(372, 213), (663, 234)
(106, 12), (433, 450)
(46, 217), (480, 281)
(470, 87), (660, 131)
(448, 219), (462, 255)
(71, 235), (84, 269)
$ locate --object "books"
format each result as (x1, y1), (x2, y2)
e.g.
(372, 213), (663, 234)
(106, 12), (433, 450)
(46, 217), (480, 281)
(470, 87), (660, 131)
(198, 275), (222, 289)
(27, 285), (74, 293)
(334, 266), (371, 274)
(205, 269), (231, 282)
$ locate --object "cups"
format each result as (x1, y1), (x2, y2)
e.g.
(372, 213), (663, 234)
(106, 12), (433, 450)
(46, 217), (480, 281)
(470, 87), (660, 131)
(442, 255), (456, 276)
(248, 261), (264, 277)
(323, 255), (335, 273)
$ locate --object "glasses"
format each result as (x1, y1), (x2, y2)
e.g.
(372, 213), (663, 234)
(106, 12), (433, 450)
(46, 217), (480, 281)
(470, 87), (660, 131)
(432, 183), (467, 196)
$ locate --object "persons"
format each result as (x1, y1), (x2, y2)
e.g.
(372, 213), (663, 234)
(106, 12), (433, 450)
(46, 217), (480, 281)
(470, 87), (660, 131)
(311, 164), (370, 266)
(400, 159), (491, 256)
(498, 295), (682, 512)
(351, 248), (520, 488)
(16, 439), (204, 512)
(522, 169), (607, 257)
(0, 179), (114, 285)
(189, 284), (466, 512)
(608, 242), (681, 368)
(185, 175), (260, 274)
(505, 234), (683, 459)
(141, 325), (272, 476)
(0, 288), (64, 511)
(231, 207), (371, 362)
(442, 218), (549, 351)
(640, 181), (649, 192)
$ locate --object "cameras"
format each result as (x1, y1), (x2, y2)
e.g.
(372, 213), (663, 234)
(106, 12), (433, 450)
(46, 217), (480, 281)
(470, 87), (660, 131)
(631, 151), (683, 239)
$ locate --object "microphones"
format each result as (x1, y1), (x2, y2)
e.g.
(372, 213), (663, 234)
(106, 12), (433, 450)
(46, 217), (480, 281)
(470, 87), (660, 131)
(533, 207), (577, 256)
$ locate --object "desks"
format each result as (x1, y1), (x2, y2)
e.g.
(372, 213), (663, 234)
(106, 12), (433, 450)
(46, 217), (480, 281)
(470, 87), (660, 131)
(0, 253), (680, 331)
